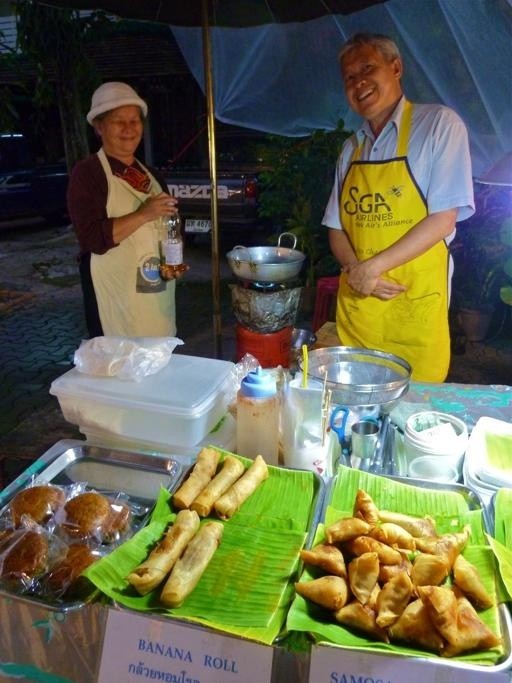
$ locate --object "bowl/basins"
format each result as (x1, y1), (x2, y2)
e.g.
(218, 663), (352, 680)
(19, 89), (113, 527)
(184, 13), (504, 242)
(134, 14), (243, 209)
(286, 360), (411, 422)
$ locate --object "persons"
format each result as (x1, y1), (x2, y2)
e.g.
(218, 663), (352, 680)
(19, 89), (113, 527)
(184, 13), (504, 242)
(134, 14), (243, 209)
(321, 30), (477, 382)
(69, 81), (193, 342)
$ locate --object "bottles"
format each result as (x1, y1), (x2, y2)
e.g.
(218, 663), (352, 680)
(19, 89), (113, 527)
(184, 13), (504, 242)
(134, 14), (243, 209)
(158, 198), (184, 274)
(235, 364), (281, 469)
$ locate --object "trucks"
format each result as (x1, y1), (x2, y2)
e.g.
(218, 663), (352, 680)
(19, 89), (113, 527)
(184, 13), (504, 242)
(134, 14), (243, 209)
(145, 127), (286, 247)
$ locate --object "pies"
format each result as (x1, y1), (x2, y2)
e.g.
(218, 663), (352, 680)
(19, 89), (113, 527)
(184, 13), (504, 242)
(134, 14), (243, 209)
(294, 488), (502, 658)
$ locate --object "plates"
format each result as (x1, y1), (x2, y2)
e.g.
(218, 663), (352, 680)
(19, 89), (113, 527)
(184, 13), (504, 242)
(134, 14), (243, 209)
(460, 412), (512, 508)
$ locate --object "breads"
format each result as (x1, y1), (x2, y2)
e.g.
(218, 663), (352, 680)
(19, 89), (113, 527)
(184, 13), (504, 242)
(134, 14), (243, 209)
(0, 485), (131, 599)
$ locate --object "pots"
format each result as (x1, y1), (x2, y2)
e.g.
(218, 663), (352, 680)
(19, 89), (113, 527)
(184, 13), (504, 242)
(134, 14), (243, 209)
(224, 231), (308, 286)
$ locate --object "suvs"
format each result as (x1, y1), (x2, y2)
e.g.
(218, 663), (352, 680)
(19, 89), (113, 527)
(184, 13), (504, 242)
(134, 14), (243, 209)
(0, 161), (69, 226)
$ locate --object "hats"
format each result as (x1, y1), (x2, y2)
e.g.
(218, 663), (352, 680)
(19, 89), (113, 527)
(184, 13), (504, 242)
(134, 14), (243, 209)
(86, 82), (148, 125)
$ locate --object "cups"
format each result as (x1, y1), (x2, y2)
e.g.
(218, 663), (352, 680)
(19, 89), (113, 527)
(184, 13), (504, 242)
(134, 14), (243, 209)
(350, 422), (380, 460)
(401, 409), (469, 481)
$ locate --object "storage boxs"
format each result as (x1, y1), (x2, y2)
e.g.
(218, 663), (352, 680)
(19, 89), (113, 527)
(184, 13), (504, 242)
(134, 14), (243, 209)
(79, 410), (238, 464)
(48, 352), (238, 449)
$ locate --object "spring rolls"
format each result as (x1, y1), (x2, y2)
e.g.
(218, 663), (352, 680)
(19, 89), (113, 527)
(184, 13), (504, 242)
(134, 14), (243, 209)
(123, 447), (269, 608)
